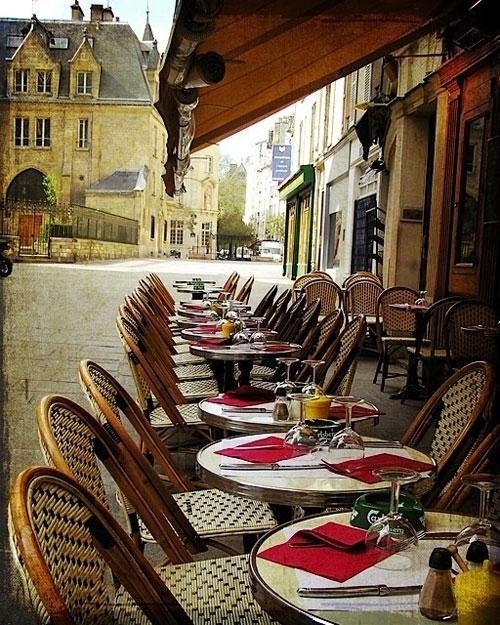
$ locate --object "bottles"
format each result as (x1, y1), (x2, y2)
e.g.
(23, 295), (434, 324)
(289, 387), (302, 421)
(454, 541), (499, 625)
(418, 546), (458, 623)
(272, 387), (290, 421)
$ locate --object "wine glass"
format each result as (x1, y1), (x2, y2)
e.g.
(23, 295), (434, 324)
(232, 317), (249, 349)
(328, 395), (365, 459)
(284, 392), (321, 453)
(216, 306), (229, 329)
(203, 290), (213, 305)
(452, 473), (500, 574)
(276, 356), (300, 397)
(364, 465), (422, 570)
(301, 358), (326, 396)
(233, 308), (247, 329)
(249, 316), (267, 348)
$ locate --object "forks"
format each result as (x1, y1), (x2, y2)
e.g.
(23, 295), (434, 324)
(320, 458), (424, 475)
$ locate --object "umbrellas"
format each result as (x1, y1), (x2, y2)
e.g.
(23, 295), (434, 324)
(156, 10), (491, 199)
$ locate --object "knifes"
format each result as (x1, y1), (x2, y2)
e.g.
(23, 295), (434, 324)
(295, 582), (429, 598)
(219, 462), (327, 470)
(221, 407), (274, 413)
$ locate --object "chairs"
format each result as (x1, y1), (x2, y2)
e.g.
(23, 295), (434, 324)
(308, 312), (367, 394)
(36, 393), (281, 625)
(7, 466), (195, 625)
(293, 271), (341, 315)
(253, 284), (278, 317)
(218, 270), (240, 300)
(274, 292), (307, 340)
(116, 272), (228, 432)
(235, 274), (254, 305)
(400, 295), (499, 404)
(373, 286), (432, 392)
(400, 360), (492, 489)
(78, 359), (278, 566)
(233, 297), (322, 380)
(342, 271), (385, 320)
(259, 287), (293, 330)
(237, 309), (344, 392)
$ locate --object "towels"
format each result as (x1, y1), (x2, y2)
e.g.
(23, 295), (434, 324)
(188, 316), (207, 323)
(255, 521), (408, 584)
(185, 324), (219, 335)
(329, 403), (386, 419)
(189, 337), (230, 347)
(185, 304), (206, 313)
(266, 343), (291, 351)
(324, 452), (436, 485)
(203, 385), (275, 407)
(213, 436), (318, 463)
